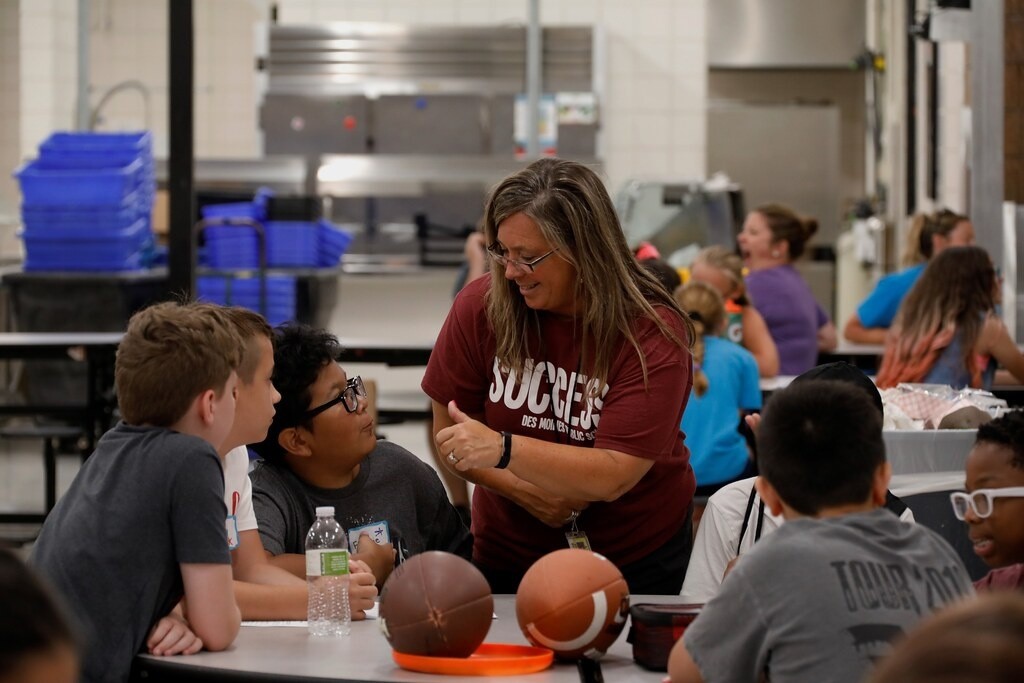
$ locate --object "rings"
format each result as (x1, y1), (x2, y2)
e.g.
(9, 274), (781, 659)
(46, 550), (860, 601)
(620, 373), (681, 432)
(447, 451), (458, 465)
(565, 510), (578, 521)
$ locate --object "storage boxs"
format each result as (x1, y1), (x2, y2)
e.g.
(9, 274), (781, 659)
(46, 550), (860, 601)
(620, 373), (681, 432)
(884, 430), (978, 471)
(11, 131), (350, 328)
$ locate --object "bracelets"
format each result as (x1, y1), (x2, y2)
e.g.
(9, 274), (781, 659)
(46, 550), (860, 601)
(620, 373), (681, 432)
(494, 430), (512, 469)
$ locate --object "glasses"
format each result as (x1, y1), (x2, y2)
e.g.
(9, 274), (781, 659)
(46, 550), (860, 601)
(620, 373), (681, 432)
(303, 375), (367, 421)
(950, 486), (1024, 520)
(487, 242), (560, 273)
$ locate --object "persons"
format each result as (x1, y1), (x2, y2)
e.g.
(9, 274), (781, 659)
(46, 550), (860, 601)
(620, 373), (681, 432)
(0, 288), (471, 683)
(420, 157), (698, 595)
(449, 217), (491, 299)
(667, 361), (1024, 683)
(631, 204), (1023, 497)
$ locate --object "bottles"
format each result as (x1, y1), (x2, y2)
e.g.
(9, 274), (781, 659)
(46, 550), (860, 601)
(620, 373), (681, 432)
(305, 506), (352, 640)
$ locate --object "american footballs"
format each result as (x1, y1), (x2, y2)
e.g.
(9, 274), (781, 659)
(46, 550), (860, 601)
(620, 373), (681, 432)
(376, 549), (495, 659)
(515, 549), (631, 660)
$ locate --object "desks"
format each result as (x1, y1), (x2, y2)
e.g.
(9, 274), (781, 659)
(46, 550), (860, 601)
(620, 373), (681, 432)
(138, 592), (690, 683)
